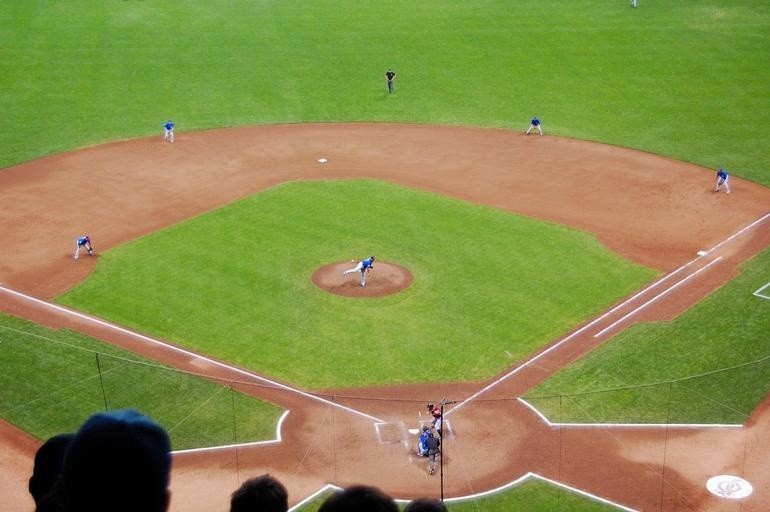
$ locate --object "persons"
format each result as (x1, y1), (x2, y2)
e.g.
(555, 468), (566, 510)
(163, 120), (175, 143)
(402, 498), (448, 512)
(318, 485), (399, 512)
(344, 256), (375, 288)
(416, 403), (455, 475)
(29, 433), (75, 507)
(385, 69), (396, 93)
(715, 169), (729, 194)
(37, 409), (172, 512)
(631, 0), (637, 8)
(526, 117), (543, 135)
(74, 236), (94, 261)
(231, 474), (288, 512)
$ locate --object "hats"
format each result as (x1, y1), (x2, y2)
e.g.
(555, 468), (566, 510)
(63, 409), (170, 482)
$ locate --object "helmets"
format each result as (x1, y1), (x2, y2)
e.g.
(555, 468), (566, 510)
(429, 433), (433, 437)
(428, 404), (433, 409)
(423, 428), (427, 431)
